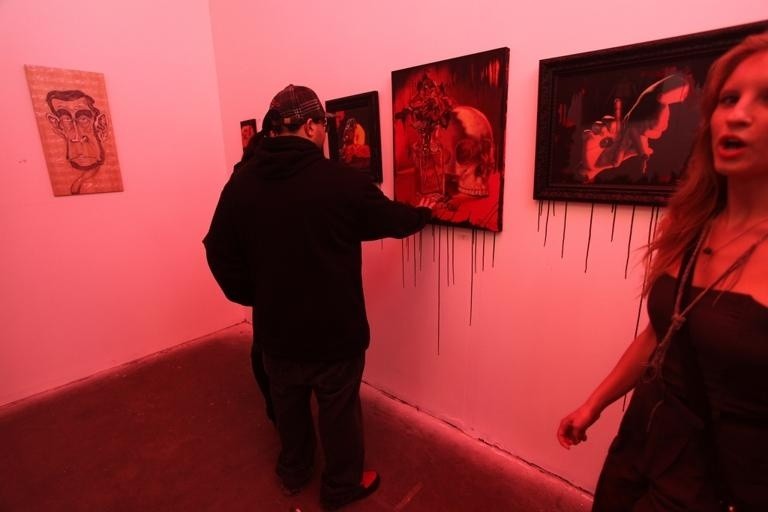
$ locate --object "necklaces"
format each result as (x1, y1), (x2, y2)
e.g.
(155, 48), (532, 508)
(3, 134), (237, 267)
(702, 217), (768, 257)
(640, 213), (767, 385)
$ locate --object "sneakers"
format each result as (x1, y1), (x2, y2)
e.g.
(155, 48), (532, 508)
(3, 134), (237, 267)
(318, 470), (380, 511)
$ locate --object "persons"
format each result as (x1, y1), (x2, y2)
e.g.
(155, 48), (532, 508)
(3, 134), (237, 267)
(45, 90), (123, 196)
(562, 73), (690, 184)
(554, 29), (768, 510)
(202, 83), (439, 509)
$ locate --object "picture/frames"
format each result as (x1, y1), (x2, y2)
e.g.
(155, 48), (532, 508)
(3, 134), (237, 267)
(533, 19), (768, 208)
(325, 90), (383, 184)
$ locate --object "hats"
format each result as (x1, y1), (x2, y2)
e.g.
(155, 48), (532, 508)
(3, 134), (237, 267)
(269, 85), (337, 126)
